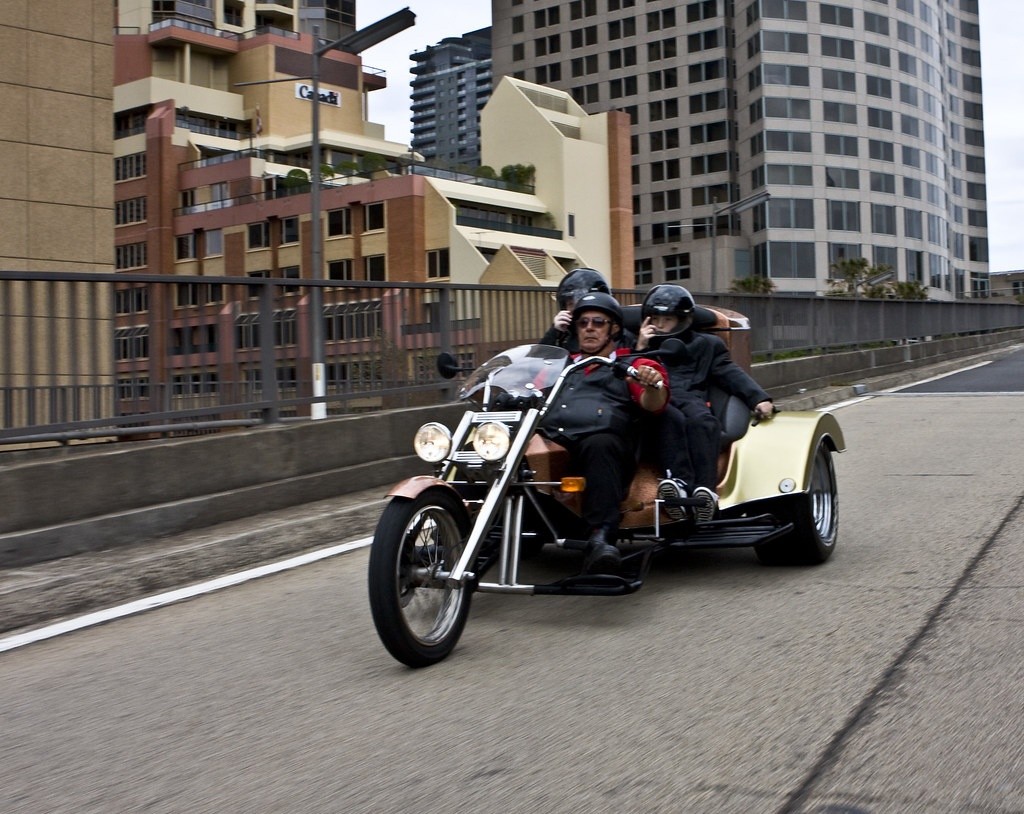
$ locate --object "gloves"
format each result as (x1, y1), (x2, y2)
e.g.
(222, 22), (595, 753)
(554, 310), (572, 333)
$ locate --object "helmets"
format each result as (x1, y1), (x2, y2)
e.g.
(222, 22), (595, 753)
(557, 268), (610, 310)
(573, 292), (625, 335)
(639, 283), (696, 345)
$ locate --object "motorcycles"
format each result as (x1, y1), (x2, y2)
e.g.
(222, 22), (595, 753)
(368, 304), (847, 668)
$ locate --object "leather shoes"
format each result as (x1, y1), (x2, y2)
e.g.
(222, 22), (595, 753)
(583, 529), (620, 575)
(415, 545), (441, 564)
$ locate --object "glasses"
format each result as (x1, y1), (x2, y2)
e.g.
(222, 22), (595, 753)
(579, 317), (614, 329)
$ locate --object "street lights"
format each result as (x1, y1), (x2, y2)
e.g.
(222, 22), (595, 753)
(711, 187), (770, 293)
(312, 6), (417, 420)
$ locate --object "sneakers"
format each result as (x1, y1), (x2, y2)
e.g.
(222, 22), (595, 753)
(691, 487), (719, 528)
(657, 469), (687, 520)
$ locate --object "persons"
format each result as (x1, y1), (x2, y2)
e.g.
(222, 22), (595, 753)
(523, 292), (670, 578)
(516, 267), (637, 385)
(632, 284), (777, 527)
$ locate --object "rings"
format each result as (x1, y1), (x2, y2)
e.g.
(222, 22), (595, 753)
(647, 367), (652, 370)
